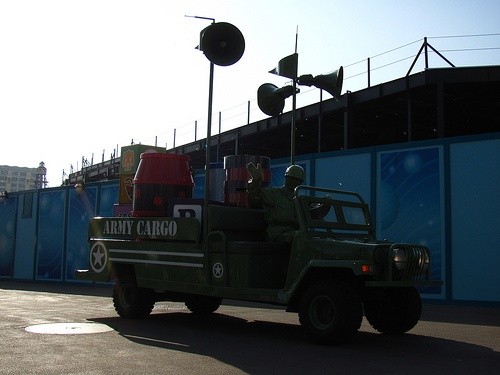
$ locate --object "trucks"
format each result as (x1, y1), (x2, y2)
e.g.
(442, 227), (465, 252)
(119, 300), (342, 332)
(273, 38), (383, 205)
(73, 185), (433, 346)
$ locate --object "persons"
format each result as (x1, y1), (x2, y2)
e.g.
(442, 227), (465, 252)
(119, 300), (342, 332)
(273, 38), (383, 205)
(245, 161), (333, 292)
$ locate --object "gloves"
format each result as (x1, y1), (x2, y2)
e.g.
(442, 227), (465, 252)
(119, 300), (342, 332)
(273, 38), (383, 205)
(247, 162), (261, 183)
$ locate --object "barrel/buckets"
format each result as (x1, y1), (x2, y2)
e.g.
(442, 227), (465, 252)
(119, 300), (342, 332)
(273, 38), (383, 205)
(132, 152), (194, 217)
(224, 155), (272, 205)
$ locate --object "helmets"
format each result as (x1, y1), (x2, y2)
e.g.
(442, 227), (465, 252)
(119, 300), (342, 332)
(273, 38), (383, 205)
(284, 165), (305, 182)
